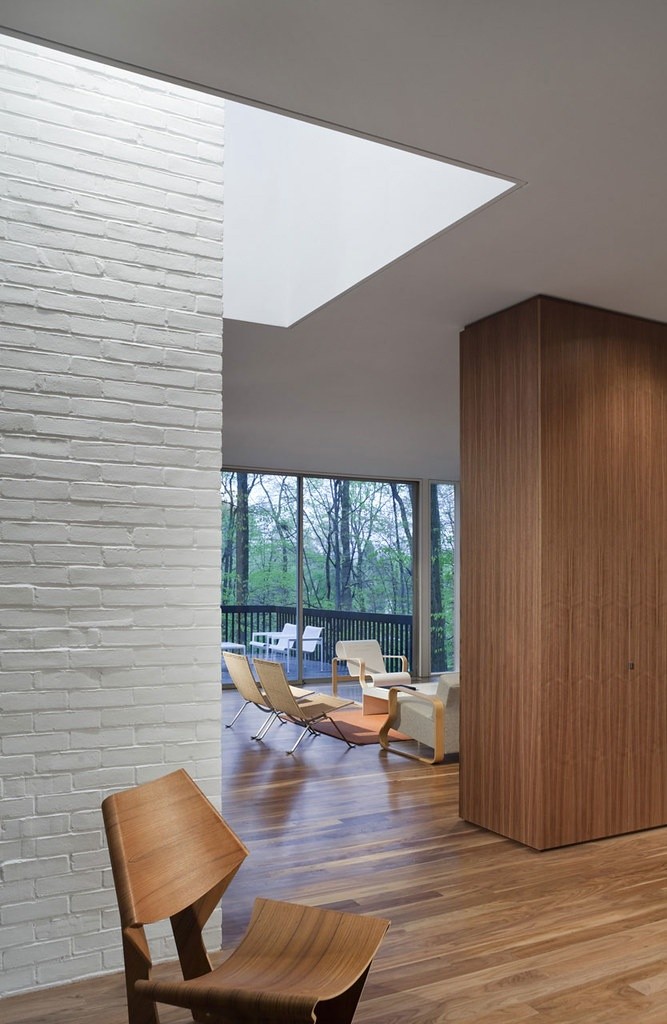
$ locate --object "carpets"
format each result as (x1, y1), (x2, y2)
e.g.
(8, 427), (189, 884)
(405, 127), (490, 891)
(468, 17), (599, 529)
(279, 708), (415, 746)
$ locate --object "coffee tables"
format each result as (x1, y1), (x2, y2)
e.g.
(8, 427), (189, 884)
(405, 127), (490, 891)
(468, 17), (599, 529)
(362, 682), (438, 716)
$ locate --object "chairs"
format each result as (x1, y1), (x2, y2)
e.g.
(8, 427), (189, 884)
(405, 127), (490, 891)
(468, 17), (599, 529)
(332, 640), (408, 706)
(102, 768), (392, 1024)
(253, 659), (356, 754)
(223, 651), (315, 740)
(221, 642), (246, 656)
(272, 626), (325, 674)
(249, 623), (297, 672)
(379, 672), (460, 764)
